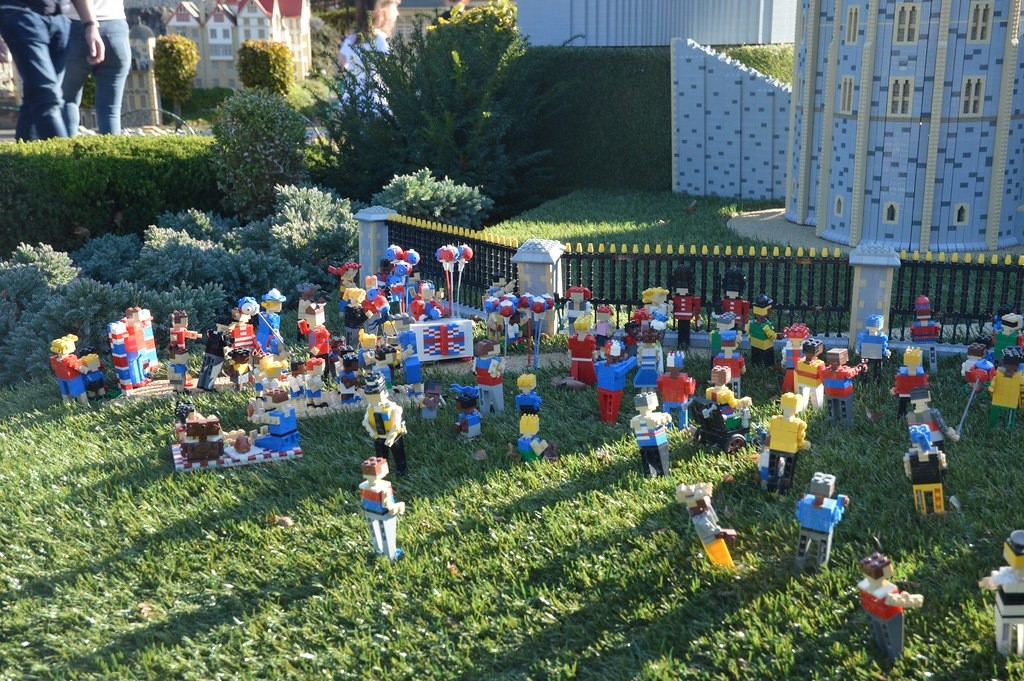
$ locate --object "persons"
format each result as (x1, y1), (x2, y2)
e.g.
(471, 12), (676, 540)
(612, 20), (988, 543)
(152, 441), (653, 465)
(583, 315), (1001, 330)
(561, 264), (892, 452)
(334, 0), (403, 124)
(890, 346), (929, 417)
(629, 391), (673, 477)
(358, 456), (406, 562)
(903, 425), (947, 514)
(754, 391), (810, 495)
(676, 483), (736, 570)
(426, 0), (470, 37)
(979, 529), (1024, 657)
(910, 295), (939, 375)
(961, 305), (1024, 429)
(794, 473), (849, 570)
(856, 553), (924, 654)
(0, 0), (131, 139)
(901, 385), (959, 450)
(49, 246), (557, 476)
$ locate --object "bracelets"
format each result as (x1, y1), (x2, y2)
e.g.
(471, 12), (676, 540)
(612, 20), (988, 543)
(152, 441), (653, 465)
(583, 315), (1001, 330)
(82, 21), (99, 29)
